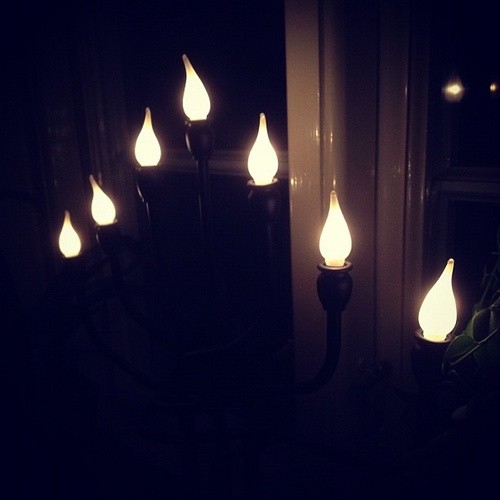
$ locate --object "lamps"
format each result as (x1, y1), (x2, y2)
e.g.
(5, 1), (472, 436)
(44, 50), (460, 395)
